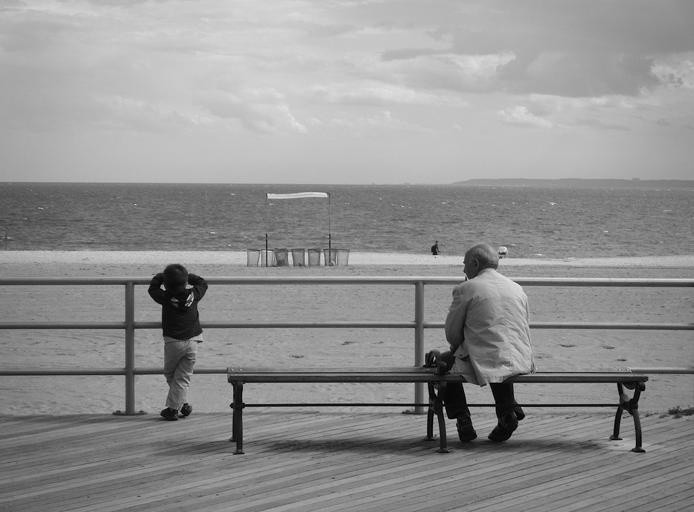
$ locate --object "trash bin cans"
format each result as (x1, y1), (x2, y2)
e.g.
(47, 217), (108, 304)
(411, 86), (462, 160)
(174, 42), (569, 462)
(247, 248), (350, 267)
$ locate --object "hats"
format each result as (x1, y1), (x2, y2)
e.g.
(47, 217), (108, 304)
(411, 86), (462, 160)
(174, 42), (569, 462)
(163, 264), (188, 295)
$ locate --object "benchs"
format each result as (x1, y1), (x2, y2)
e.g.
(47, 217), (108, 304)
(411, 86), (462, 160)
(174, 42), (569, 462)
(227, 367), (648, 455)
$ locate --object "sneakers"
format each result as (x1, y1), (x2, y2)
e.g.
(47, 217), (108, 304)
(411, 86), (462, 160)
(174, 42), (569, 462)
(456, 412), (477, 441)
(488, 405), (525, 441)
(160, 403), (192, 421)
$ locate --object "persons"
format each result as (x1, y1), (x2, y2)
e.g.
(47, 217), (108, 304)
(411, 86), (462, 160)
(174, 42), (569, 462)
(432, 242), (536, 444)
(146, 263), (209, 421)
(430, 240), (440, 256)
